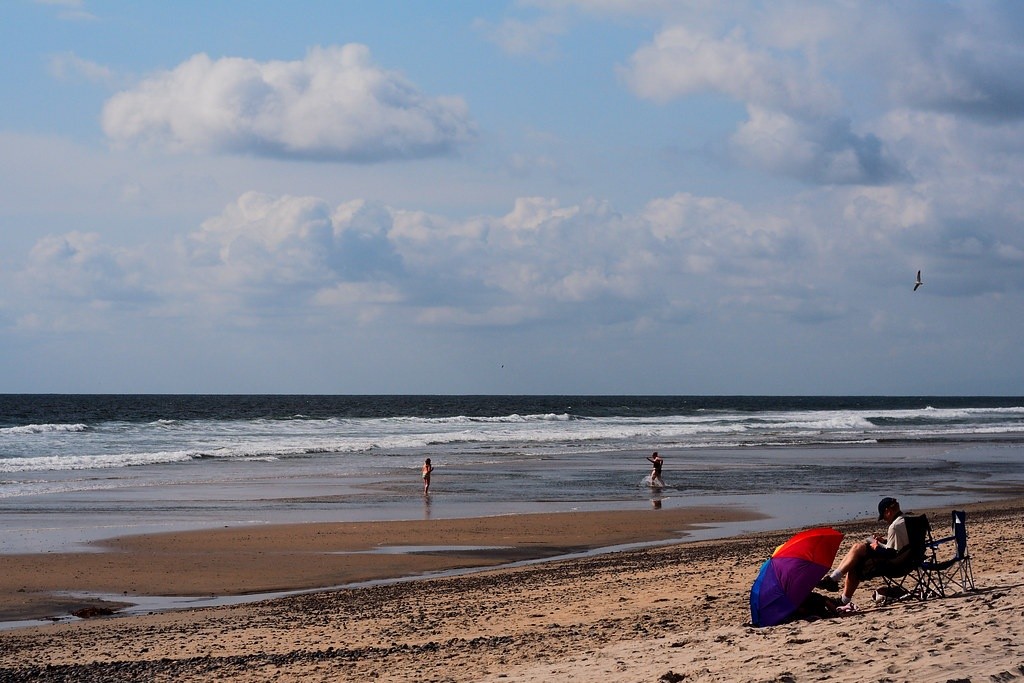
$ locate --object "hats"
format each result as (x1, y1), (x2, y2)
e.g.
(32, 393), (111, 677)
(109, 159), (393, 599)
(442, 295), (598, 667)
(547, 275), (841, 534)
(877, 497), (896, 521)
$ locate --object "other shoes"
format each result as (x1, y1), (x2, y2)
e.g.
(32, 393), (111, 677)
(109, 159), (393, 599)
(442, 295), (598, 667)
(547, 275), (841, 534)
(816, 575), (839, 591)
(835, 597), (844, 606)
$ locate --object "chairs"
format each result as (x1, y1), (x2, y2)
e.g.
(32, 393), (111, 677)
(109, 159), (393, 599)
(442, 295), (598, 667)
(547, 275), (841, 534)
(860, 513), (946, 608)
(921, 509), (975, 592)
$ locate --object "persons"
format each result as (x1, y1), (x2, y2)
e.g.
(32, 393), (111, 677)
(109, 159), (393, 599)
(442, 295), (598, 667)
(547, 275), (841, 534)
(816, 497), (908, 607)
(652, 500), (661, 509)
(646, 452), (665, 485)
(799, 592), (836, 611)
(422, 458), (434, 493)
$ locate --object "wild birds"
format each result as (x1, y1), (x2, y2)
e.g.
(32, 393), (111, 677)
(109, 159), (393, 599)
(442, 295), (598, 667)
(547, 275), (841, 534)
(913, 270), (924, 292)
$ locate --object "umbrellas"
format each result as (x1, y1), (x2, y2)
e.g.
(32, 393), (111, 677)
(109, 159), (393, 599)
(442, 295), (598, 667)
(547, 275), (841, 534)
(749, 528), (845, 627)
